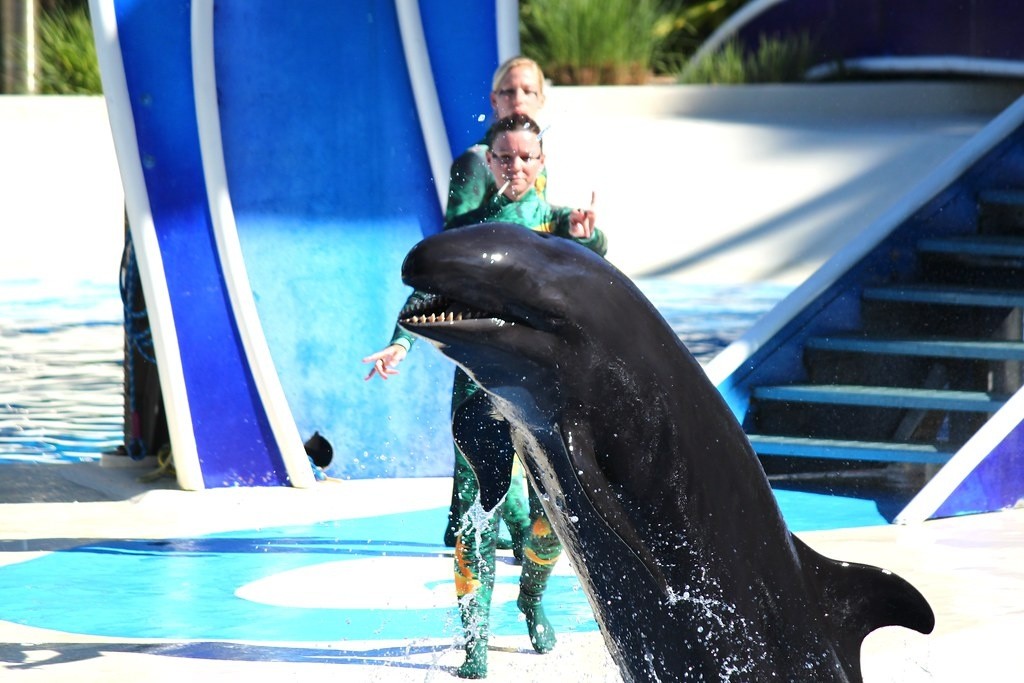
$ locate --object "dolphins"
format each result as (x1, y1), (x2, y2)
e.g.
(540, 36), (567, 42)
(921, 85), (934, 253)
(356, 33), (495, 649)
(399, 221), (934, 683)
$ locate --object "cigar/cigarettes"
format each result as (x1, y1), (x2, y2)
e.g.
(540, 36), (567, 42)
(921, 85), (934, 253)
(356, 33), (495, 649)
(493, 179), (511, 203)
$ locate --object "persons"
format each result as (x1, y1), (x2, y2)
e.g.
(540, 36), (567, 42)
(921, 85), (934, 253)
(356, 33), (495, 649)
(363, 112), (608, 679)
(444, 56), (548, 563)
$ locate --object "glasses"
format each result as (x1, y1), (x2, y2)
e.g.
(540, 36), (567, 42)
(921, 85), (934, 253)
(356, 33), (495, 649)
(488, 153), (541, 163)
(494, 89), (539, 96)
